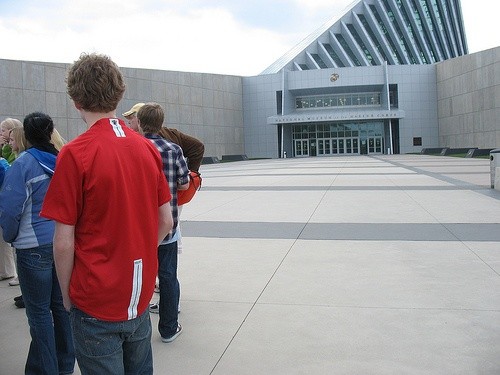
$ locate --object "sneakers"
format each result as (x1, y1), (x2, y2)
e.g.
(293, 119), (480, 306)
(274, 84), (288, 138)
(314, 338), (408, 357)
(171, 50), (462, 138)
(16, 301), (25, 307)
(150, 301), (180, 313)
(14, 295), (23, 301)
(160, 322), (183, 343)
(154, 283), (160, 293)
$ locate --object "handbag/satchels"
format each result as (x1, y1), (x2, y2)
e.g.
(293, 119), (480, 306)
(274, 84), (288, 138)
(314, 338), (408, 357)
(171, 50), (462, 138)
(177, 170), (202, 206)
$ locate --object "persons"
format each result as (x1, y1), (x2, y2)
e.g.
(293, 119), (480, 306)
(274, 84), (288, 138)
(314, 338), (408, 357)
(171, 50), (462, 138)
(39, 52), (174, 375)
(0, 112), (75, 375)
(0, 117), (66, 308)
(122, 101), (205, 342)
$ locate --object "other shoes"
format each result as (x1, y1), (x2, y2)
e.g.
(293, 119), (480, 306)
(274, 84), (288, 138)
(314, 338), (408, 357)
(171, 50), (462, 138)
(9, 277), (19, 285)
(0, 276), (4, 280)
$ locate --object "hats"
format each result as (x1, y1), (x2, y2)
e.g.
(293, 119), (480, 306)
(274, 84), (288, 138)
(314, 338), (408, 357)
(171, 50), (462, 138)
(122, 103), (145, 117)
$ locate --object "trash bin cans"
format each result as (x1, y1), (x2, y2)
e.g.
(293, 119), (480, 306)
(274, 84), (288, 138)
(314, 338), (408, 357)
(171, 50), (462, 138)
(388, 148), (390, 155)
(284, 152), (286, 158)
(490, 148), (500, 191)
